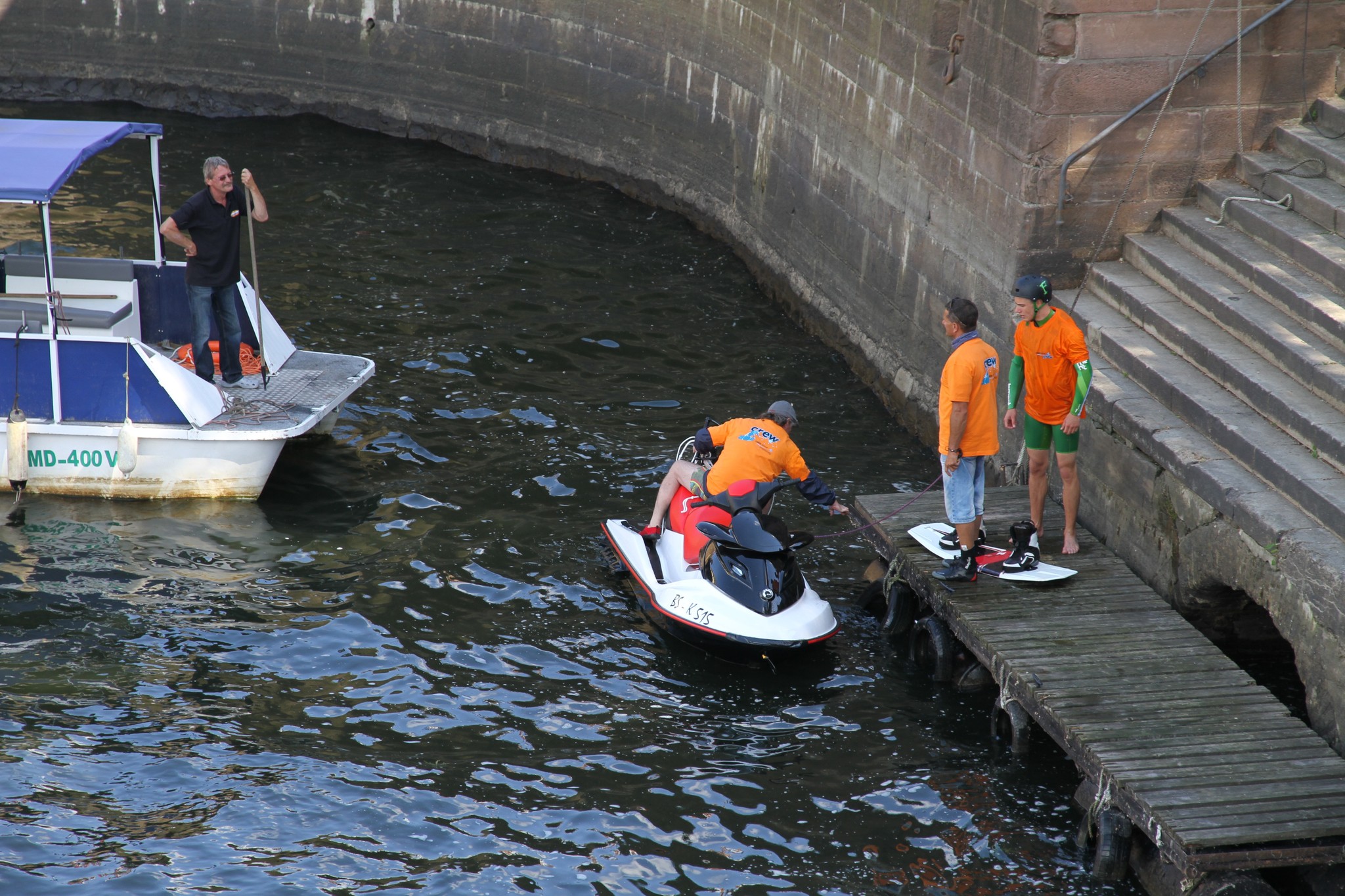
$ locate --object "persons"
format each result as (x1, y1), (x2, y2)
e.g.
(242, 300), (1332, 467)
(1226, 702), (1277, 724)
(638, 401), (849, 540)
(159, 156), (268, 389)
(930, 297), (1000, 583)
(1003, 274), (1093, 554)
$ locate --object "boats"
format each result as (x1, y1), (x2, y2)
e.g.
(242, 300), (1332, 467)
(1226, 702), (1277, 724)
(1, 118), (376, 505)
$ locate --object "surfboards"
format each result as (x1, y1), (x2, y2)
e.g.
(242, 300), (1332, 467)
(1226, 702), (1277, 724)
(907, 523), (1078, 583)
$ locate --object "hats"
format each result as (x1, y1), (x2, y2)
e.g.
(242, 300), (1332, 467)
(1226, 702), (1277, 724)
(767, 400), (799, 428)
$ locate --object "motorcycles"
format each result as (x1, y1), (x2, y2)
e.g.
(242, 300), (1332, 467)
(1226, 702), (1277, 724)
(601, 415), (841, 668)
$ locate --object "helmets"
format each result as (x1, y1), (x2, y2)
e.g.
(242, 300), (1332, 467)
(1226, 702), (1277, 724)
(1011, 274), (1052, 303)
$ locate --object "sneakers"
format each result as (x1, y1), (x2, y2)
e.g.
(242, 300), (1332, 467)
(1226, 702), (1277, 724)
(942, 555), (961, 568)
(638, 525), (662, 539)
(932, 564), (977, 582)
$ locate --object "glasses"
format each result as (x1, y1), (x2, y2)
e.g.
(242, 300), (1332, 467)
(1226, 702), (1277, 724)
(946, 297), (968, 327)
(211, 172), (235, 181)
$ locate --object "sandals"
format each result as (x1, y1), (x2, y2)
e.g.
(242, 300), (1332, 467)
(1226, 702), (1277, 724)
(221, 376), (259, 389)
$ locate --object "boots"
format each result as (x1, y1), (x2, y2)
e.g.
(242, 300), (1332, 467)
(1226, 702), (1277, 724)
(1002, 519), (1041, 572)
(939, 516), (986, 549)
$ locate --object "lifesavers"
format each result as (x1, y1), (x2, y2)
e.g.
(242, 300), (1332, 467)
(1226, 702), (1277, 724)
(904, 612), (957, 686)
(990, 695), (1036, 756)
(178, 340), (254, 365)
(853, 577), (917, 637)
(1076, 802), (1134, 883)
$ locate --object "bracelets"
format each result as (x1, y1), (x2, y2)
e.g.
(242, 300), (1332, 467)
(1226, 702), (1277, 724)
(947, 449), (958, 453)
